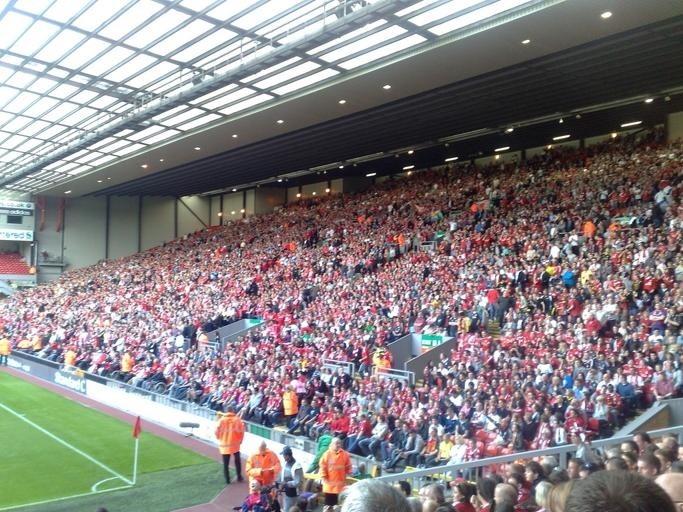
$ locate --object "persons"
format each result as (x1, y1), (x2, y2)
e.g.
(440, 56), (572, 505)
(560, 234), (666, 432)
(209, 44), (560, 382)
(214, 405), (244, 486)
(0, 219), (236, 512)
(238, 133), (683, 511)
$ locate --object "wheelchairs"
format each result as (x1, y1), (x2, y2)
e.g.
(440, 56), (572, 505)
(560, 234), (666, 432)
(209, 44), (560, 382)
(232, 483), (287, 512)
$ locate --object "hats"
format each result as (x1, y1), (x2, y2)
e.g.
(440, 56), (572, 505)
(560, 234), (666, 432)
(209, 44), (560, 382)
(405, 428), (416, 436)
(278, 446), (292, 456)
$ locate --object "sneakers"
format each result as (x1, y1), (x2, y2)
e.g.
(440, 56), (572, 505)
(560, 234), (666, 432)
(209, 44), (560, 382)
(368, 454), (395, 473)
